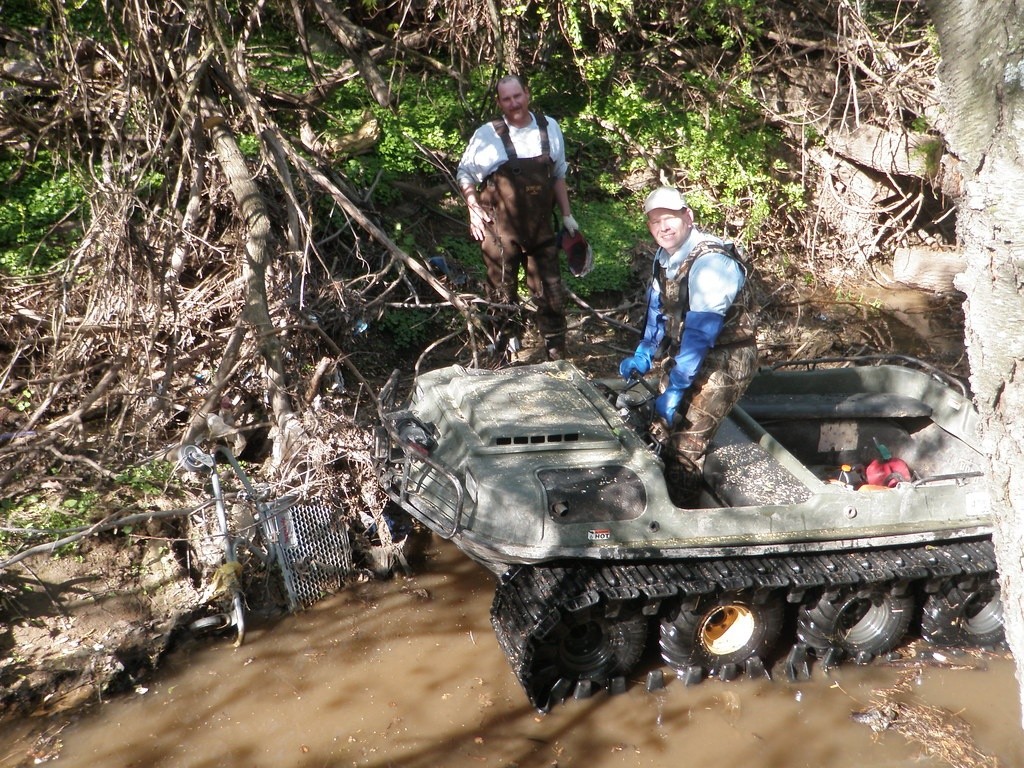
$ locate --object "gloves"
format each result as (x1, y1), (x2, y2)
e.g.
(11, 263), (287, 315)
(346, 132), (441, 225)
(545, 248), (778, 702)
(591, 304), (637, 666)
(619, 286), (671, 384)
(563, 214), (579, 238)
(655, 310), (723, 430)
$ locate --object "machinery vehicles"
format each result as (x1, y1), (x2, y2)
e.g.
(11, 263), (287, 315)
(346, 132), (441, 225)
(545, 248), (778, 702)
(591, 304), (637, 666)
(371, 363), (1008, 717)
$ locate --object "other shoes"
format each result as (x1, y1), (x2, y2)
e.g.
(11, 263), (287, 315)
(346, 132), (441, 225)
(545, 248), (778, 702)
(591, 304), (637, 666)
(544, 336), (565, 361)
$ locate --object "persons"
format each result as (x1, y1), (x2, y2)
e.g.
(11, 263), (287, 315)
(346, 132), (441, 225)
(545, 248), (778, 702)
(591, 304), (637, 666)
(619, 187), (759, 508)
(455, 75), (579, 362)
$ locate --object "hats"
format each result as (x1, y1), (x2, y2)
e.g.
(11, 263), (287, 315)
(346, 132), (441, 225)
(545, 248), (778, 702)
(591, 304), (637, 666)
(643, 186), (693, 216)
(562, 229), (593, 278)
